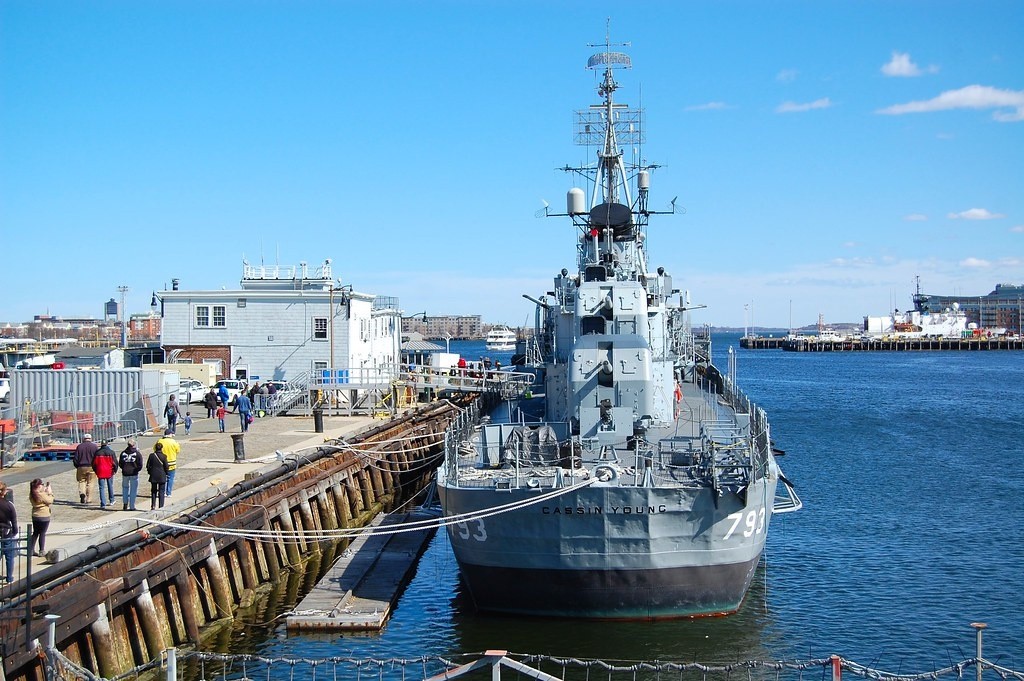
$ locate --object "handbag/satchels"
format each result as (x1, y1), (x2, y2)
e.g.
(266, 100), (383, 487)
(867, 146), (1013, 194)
(246, 416), (254, 423)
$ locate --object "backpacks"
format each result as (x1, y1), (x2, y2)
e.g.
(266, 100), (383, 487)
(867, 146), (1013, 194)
(167, 401), (176, 416)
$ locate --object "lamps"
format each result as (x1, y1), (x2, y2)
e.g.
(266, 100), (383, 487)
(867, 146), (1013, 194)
(337, 278), (342, 283)
(340, 292), (350, 305)
(222, 286), (225, 290)
(238, 299), (246, 307)
(402, 311), (428, 323)
(151, 292), (162, 305)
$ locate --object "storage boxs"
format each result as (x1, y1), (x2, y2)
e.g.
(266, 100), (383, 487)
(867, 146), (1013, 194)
(671, 449), (701, 465)
(9, 363), (216, 432)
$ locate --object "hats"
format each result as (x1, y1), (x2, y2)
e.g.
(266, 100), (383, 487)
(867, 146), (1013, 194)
(165, 429), (175, 435)
(101, 439), (109, 446)
(83, 433), (91, 439)
(128, 439), (137, 447)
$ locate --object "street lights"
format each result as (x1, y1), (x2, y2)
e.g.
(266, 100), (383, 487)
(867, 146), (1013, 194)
(750, 298), (755, 337)
(789, 299), (792, 334)
(116, 284), (128, 348)
(328, 284), (353, 382)
(392, 311), (428, 375)
(1019, 297), (1022, 337)
(979, 296), (982, 327)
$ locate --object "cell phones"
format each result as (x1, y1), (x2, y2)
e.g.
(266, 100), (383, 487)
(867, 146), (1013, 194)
(47, 482), (49, 486)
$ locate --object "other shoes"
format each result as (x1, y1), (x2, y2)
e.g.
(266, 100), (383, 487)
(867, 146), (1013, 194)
(31, 549), (46, 557)
(123, 503), (136, 511)
(100, 500), (116, 510)
(80, 494), (93, 503)
(242, 429), (248, 433)
(219, 429), (225, 433)
(185, 431), (190, 435)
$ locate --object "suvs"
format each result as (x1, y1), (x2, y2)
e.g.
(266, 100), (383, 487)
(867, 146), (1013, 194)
(210, 379), (248, 406)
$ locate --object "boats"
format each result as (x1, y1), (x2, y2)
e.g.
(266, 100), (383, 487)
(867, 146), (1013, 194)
(893, 273), (967, 337)
(484, 321), (518, 351)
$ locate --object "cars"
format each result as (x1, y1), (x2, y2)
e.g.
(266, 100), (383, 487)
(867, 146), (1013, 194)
(0, 377), (10, 402)
(248, 379), (302, 401)
(179, 379), (211, 404)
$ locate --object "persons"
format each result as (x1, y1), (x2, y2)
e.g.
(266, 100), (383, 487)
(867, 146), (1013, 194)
(401, 354), (437, 375)
(205, 389), (218, 419)
(180, 412), (193, 435)
(73, 433), (98, 503)
(152, 429), (181, 498)
(219, 382), (230, 415)
(0, 482), (18, 582)
(146, 443), (169, 510)
(450, 356), (500, 379)
(29, 478), (54, 557)
(244, 381), (276, 416)
(92, 439), (123, 509)
(164, 395), (181, 437)
(118, 439), (143, 511)
(217, 404), (233, 433)
(454, 390), (479, 404)
(232, 390), (252, 432)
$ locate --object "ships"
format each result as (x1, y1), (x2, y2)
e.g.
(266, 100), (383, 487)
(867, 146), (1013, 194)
(434, 16), (803, 621)
